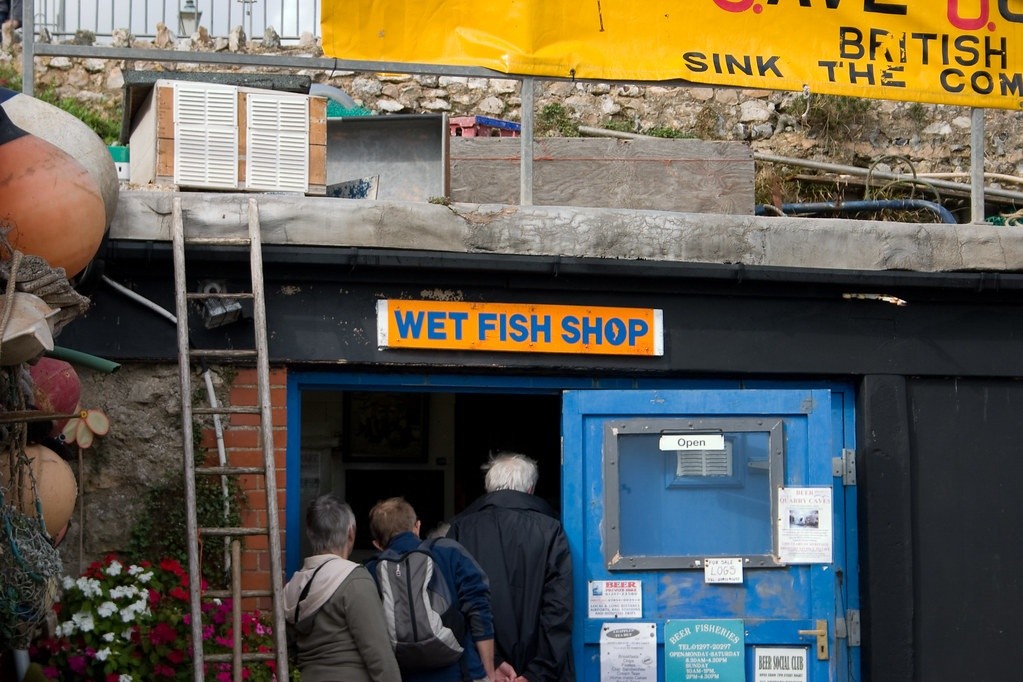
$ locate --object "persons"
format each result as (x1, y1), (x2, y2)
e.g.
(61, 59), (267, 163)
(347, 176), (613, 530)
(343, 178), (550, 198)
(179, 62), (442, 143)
(444, 452), (574, 682)
(364, 497), (495, 682)
(282, 494), (402, 682)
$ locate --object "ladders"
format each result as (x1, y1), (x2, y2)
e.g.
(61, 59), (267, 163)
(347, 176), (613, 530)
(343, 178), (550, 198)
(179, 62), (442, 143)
(172, 197), (290, 682)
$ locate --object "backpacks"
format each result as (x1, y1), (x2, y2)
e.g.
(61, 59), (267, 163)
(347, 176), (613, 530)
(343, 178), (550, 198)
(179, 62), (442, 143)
(372, 537), (466, 676)
